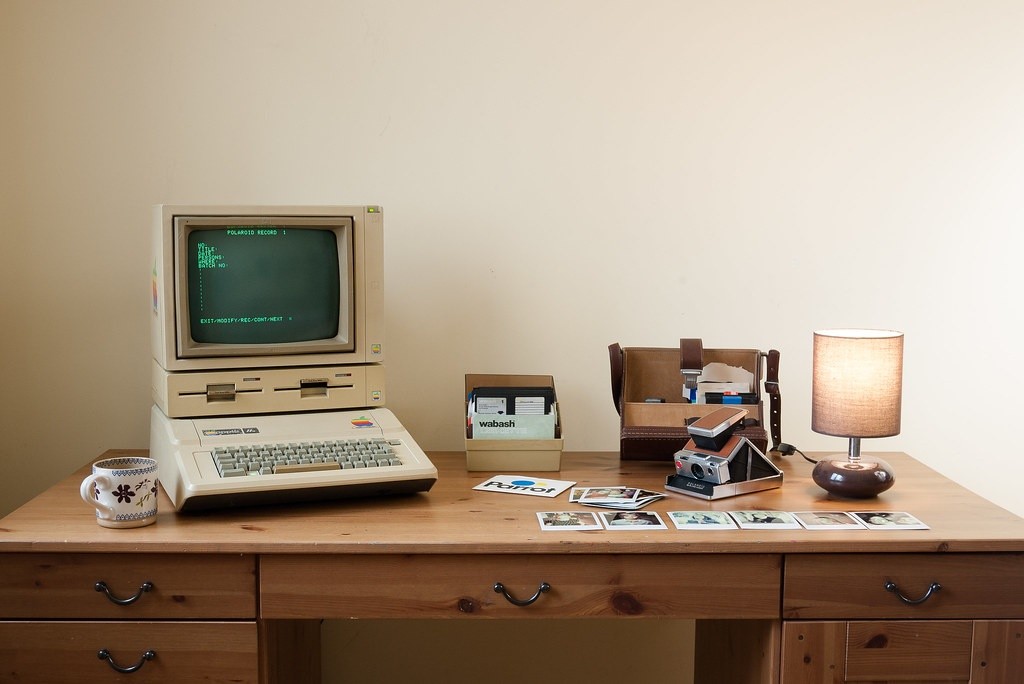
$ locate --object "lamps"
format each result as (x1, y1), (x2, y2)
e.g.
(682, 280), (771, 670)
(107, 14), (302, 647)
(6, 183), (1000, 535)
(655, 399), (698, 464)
(807, 332), (904, 497)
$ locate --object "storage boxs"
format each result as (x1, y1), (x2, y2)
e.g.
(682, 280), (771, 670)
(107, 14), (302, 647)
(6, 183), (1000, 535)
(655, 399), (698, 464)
(624, 346), (762, 427)
(464, 371), (564, 470)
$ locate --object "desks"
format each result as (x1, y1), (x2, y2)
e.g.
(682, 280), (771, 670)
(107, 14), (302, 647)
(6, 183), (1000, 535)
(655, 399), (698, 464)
(0, 449), (1024, 684)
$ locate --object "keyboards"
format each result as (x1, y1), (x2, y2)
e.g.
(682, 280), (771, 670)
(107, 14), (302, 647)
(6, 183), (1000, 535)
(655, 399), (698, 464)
(211, 438), (402, 478)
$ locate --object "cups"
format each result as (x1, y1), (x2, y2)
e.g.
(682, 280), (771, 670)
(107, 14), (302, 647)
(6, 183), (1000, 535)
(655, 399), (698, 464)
(80, 457), (157, 529)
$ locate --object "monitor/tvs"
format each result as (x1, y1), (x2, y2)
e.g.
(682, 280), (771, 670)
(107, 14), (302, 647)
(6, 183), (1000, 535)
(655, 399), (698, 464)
(151, 203), (387, 375)
